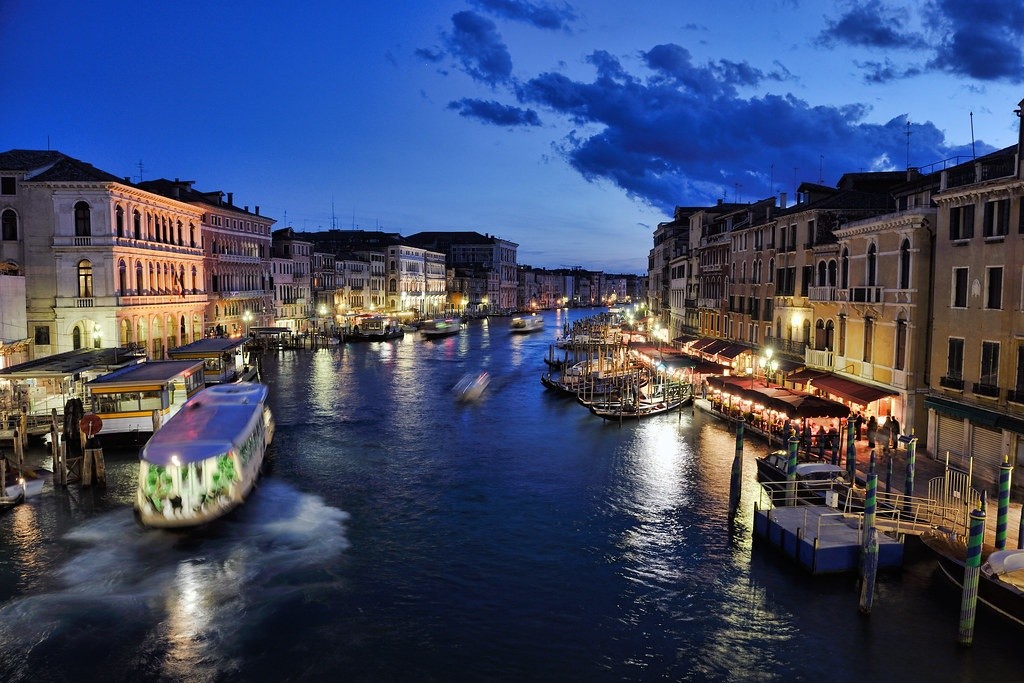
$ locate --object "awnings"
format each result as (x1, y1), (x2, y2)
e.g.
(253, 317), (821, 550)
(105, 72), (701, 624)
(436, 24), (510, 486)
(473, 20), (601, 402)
(671, 335), (750, 360)
(787, 369), (898, 406)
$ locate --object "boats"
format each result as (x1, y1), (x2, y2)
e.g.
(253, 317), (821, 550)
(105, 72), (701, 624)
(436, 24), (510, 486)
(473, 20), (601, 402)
(131, 380), (276, 530)
(920, 522), (1024, 629)
(507, 315), (545, 333)
(539, 326), (691, 422)
(755, 447), (849, 508)
(419, 317), (459, 337)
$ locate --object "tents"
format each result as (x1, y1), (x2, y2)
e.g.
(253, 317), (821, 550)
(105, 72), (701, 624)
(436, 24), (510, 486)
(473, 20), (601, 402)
(250, 326), (292, 347)
(707, 374), (851, 449)
(0, 346), (146, 413)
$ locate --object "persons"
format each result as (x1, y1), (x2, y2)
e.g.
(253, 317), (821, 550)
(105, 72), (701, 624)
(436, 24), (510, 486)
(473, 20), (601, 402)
(701, 380), (707, 399)
(783, 411), (900, 449)
